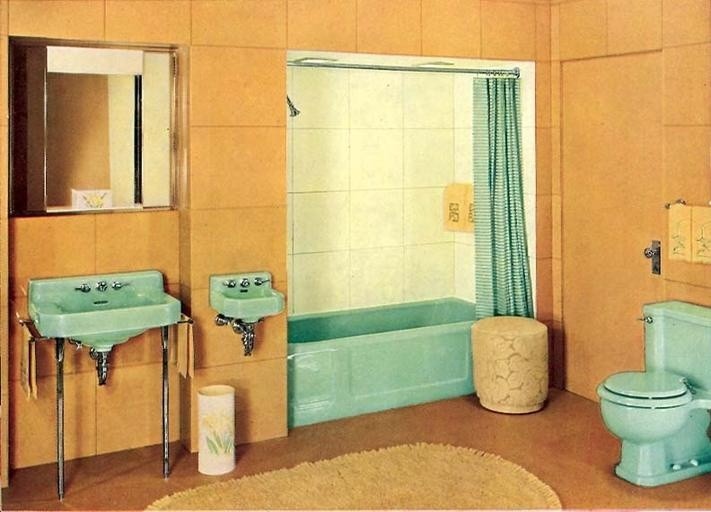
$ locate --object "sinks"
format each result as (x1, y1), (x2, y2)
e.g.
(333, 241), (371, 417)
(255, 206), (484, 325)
(208, 271), (285, 323)
(26, 268), (182, 353)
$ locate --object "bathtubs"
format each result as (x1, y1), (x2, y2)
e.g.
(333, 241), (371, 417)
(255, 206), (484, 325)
(285, 296), (485, 429)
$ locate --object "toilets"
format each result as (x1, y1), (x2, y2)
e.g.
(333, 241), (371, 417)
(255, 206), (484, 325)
(594, 300), (711, 488)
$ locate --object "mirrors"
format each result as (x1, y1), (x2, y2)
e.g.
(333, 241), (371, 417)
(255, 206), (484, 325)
(8, 35), (183, 217)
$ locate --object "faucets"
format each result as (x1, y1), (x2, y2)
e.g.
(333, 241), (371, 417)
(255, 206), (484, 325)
(239, 278), (250, 288)
(95, 280), (108, 293)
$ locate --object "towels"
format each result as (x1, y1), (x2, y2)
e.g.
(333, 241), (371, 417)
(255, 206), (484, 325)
(668, 205), (711, 264)
(19, 325), (39, 398)
(171, 313), (196, 378)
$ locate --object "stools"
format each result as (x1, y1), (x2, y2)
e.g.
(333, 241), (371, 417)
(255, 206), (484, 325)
(471, 315), (551, 413)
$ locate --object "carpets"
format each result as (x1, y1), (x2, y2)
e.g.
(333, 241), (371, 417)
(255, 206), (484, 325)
(145, 440), (564, 510)
